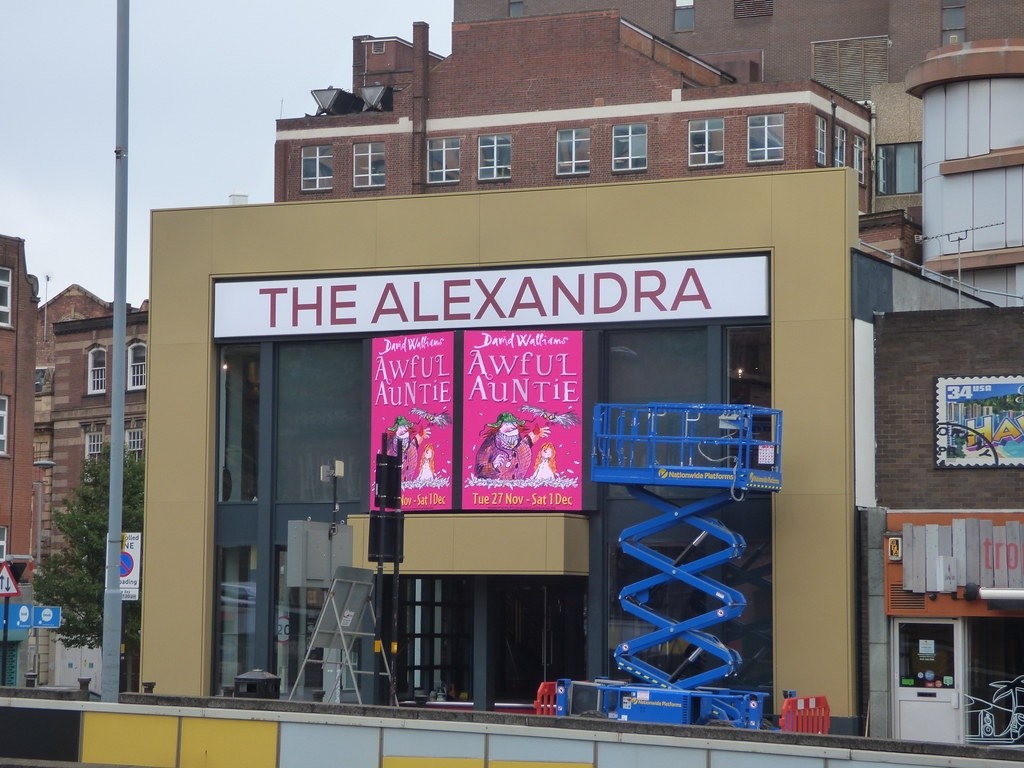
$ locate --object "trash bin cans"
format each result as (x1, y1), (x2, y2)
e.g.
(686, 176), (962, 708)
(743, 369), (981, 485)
(235, 668), (282, 700)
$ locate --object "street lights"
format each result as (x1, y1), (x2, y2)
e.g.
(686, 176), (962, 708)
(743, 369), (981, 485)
(32, 459), (57, 575)
